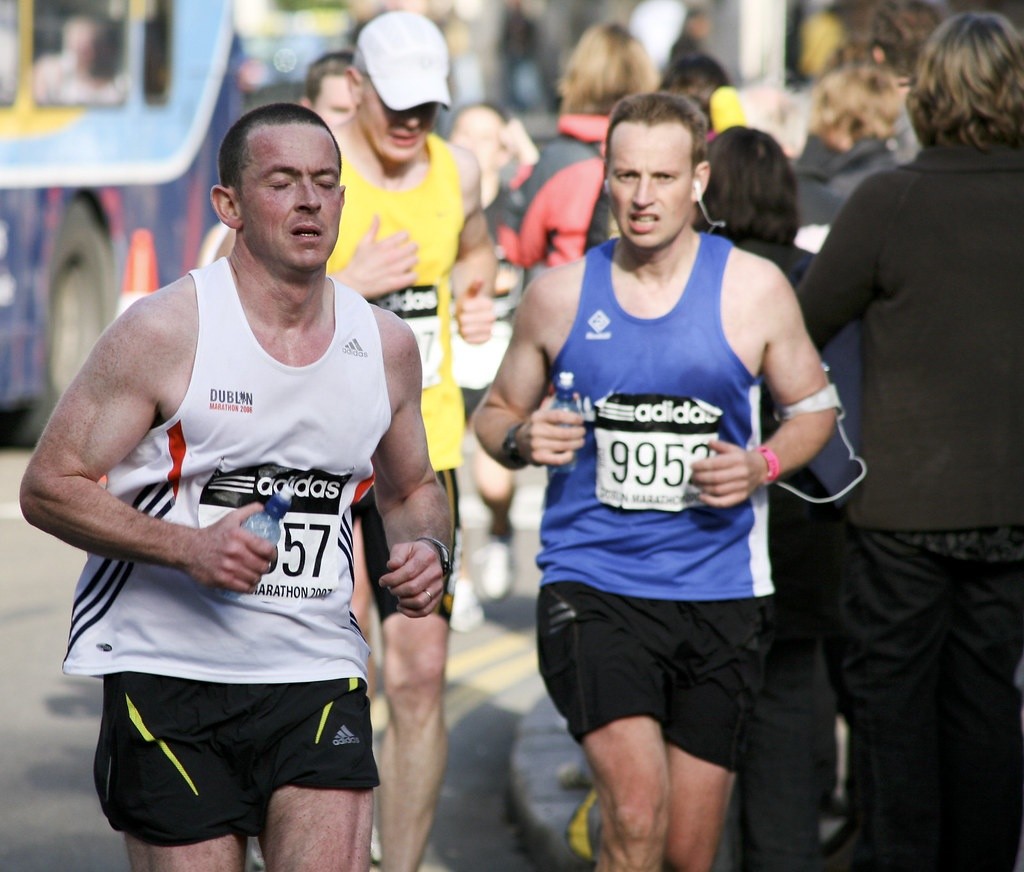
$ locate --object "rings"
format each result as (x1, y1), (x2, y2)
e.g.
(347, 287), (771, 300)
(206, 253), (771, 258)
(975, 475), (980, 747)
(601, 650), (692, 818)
(424, 591), (433, 600)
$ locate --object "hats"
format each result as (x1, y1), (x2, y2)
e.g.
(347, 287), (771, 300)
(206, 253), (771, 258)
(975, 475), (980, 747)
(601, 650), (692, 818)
(356, 12), (453, 112)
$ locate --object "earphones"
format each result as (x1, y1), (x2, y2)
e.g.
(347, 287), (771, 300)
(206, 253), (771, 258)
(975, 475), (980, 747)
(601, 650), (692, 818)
(604, 179), (609, 193)
(692, 179), (702, 201)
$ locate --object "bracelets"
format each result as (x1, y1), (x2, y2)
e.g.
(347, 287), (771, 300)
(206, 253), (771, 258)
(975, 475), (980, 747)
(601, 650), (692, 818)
(760, 447), (779, 486)
(417, 535), (452, 575)
(502, 425), (527, 469)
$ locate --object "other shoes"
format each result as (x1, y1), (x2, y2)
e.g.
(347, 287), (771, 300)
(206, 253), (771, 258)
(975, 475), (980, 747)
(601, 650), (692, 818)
(447, 576), (485, 632)
(562, 787), (604, 861)
(484, 526), (516, 597)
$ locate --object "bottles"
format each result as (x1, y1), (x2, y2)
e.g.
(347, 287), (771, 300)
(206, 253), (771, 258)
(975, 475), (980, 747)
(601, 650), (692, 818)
(203, 484), (295, 598)
(549, 372), (580, 472)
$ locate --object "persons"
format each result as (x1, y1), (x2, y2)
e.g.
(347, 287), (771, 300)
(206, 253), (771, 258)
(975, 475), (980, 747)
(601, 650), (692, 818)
(796, 14), (1023, 872)
(870, 0), (948, 166)
(443, 101), (525, 634)
(695, 121), (865, 871)
(218, 9), (501, 872)
(305, 53), (359, 127)
(526, 25), (663, 216)
(19, 106), (453, 872)
(664, 49), (728, 136)
(514, 155), (605, 270)
(791, 66), (902, 233)
(38, 14), (125, 101)
